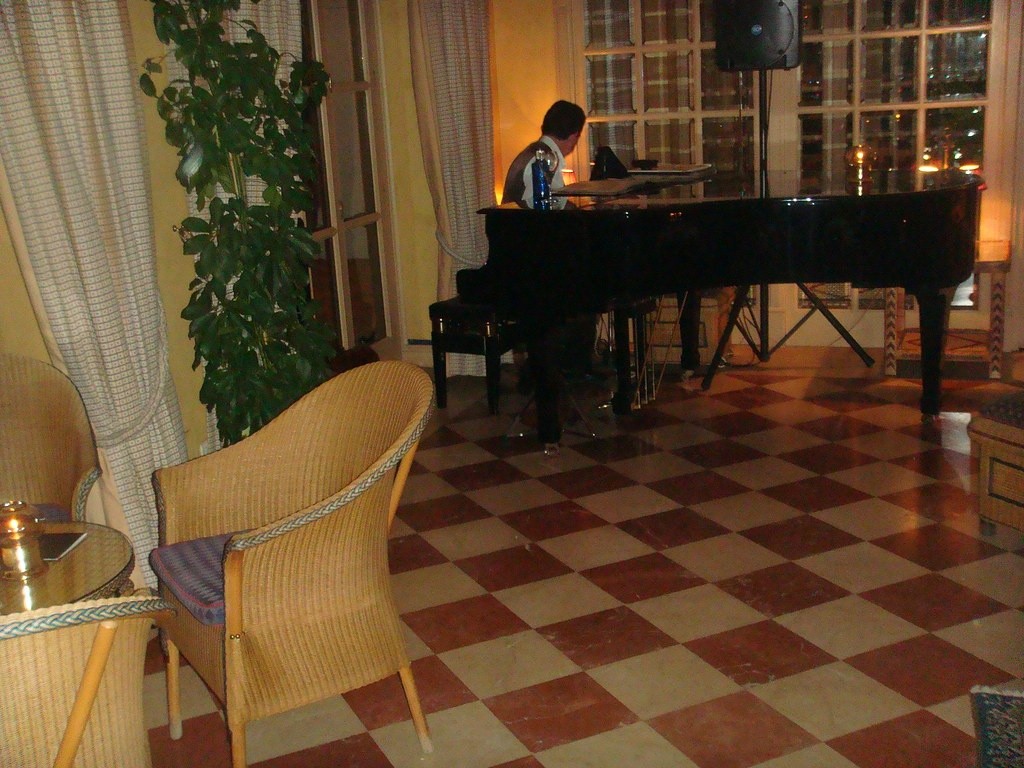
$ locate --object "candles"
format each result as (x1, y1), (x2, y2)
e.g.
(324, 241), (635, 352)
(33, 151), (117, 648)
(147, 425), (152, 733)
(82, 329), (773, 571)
(0, 500), (49, 582)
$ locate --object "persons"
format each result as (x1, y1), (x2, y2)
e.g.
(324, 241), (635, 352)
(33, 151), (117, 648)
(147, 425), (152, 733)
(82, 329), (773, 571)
(501, 100), (607, 389)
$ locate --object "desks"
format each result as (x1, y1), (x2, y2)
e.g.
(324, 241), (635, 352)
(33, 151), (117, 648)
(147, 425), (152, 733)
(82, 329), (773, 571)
(0, 521), (135, 615)
(882, 240), (1011, 380)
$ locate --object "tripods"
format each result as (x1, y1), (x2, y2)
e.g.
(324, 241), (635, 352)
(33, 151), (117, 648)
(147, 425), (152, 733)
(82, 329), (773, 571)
(701, 69), (875, 391)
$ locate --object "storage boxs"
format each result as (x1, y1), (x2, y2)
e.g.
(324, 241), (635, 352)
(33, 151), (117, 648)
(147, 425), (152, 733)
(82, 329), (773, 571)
(646, 287), (731, 362)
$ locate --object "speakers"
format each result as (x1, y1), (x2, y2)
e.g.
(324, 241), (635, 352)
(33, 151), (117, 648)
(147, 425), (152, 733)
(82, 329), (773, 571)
(715, 0), (802, 73)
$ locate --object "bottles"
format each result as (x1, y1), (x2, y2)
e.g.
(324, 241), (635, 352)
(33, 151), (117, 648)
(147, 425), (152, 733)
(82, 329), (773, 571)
(532, 149), (551, 207)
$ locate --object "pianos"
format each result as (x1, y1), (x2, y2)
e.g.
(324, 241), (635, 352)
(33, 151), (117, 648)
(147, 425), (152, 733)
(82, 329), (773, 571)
(474, 166), (989, 451)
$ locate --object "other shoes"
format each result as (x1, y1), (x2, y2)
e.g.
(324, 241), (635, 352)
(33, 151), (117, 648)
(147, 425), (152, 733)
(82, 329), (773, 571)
(562, 366), (607, 386)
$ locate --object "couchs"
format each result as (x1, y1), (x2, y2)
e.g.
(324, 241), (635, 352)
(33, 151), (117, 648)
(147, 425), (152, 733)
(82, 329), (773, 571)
(965, 385), (1024, 537)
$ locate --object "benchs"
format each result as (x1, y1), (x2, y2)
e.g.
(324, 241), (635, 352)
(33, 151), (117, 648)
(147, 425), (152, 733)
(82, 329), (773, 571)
(429, 296), (602, 416)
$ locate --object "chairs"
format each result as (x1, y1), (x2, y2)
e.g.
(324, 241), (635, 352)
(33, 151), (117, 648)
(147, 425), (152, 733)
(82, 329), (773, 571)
(0, 586), (179, 768)
(149, 359), (435, 768)
(0, 353), (104, 521)
